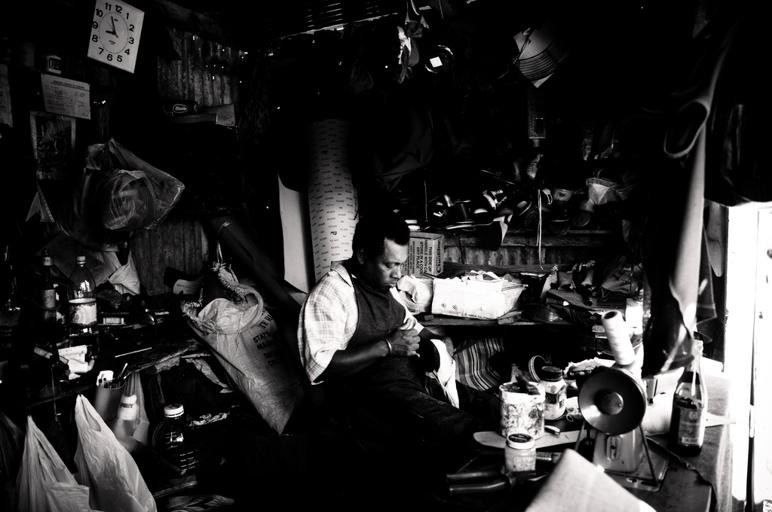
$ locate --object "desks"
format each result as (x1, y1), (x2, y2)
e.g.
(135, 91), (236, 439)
(2, 340), (208, 512)
(417, 307), (731, 512)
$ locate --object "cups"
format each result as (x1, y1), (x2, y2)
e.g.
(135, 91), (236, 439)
(537, 365), (567, 419)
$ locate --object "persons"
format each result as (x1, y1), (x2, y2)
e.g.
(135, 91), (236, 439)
(297, 210), (499, 446)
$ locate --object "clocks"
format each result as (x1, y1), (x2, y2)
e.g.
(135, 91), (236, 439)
(85, 4), (147, 78)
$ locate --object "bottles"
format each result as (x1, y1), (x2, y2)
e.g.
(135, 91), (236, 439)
(39, 248), (98, 336)
(505, 433), (536, 477)
(670, 331), (707, 457)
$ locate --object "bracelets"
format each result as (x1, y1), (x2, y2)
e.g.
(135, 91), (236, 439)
(385, 337), (393, 355)
